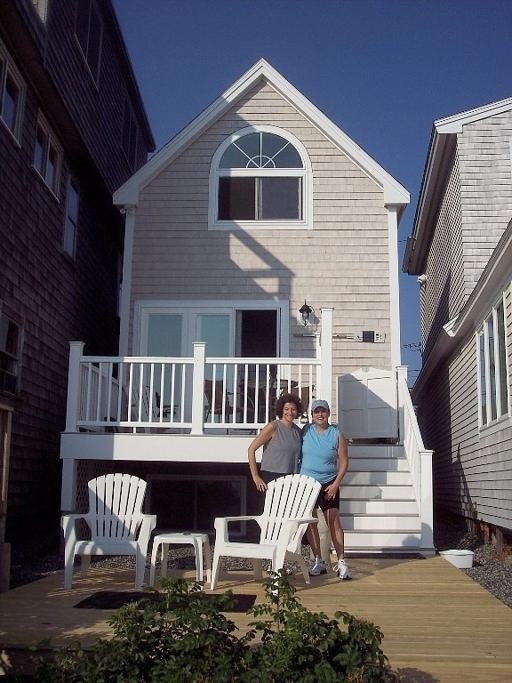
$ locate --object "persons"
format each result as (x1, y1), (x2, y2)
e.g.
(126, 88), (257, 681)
(248, 393), (352, 580)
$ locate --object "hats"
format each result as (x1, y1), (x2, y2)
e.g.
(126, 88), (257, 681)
(310, 399), (330, 411)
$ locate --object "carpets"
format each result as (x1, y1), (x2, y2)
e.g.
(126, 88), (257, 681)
(73, 591), (257, 613)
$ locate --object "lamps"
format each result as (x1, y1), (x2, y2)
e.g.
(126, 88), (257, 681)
(299, 300), (312, 327)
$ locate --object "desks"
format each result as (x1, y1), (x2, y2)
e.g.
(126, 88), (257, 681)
(245, 378), (298, 435)
(148, 532), (210, 590)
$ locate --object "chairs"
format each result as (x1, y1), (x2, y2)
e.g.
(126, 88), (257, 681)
(283, 385), (315, 428)
(203, 379), (254, 434)
(211, 473), (322, 598)
(59, 472), (157, 591)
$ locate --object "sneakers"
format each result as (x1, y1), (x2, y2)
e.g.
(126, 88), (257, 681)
(337, 560), (353, 580)
(308, 559), (327, 576)
